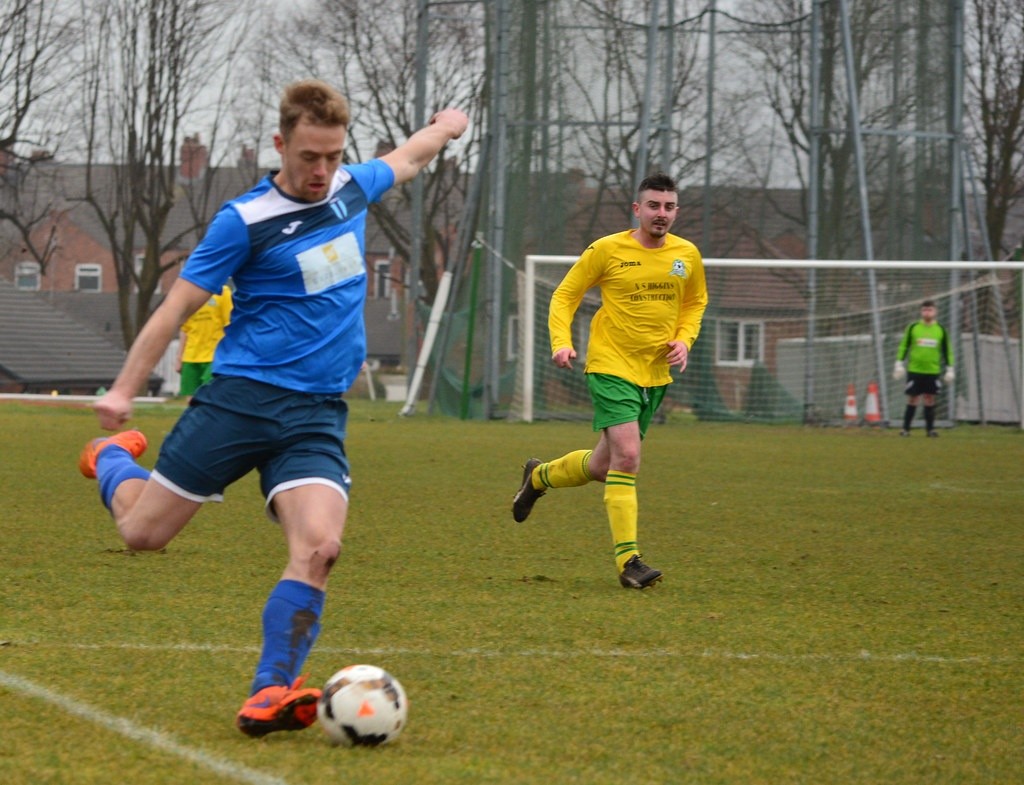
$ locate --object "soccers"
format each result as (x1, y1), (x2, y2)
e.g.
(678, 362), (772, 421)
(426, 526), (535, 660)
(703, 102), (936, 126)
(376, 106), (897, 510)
(316, 662), (408, 745)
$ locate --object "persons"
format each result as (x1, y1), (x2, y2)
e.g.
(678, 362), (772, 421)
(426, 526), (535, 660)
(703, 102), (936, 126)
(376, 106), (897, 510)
(81, 84), (469, 737)
(176, 286), (233, 402)
(893, 301), (954, 437)
(514, 174), (708, 589)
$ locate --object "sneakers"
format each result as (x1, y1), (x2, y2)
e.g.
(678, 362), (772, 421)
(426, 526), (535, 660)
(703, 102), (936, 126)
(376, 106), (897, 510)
(619, 553), (663, 589)
(79, 431), (147, 479)
(511, 457), (547, 522)
(236, 672), (323, 737)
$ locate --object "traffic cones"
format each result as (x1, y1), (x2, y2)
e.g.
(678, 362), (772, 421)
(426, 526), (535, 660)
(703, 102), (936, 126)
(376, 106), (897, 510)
(864, 382), (880, 429)
(843, 383), (858, 426)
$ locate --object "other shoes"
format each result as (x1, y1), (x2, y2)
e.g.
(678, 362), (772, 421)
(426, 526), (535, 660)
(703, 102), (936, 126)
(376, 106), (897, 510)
(900, 431), (908, 435)
(926, 432), (936, 437)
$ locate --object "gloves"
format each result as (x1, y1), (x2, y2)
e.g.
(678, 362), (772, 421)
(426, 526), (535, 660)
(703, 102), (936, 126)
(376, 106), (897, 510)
(944, 368), (954, 380)
(893, 361), (905, 379)
